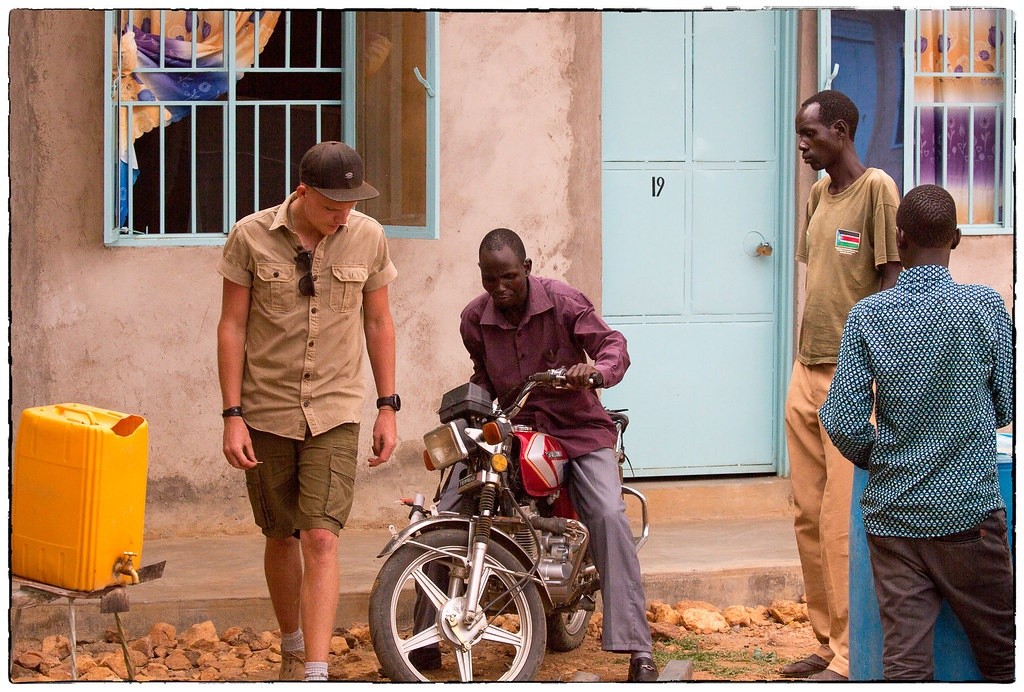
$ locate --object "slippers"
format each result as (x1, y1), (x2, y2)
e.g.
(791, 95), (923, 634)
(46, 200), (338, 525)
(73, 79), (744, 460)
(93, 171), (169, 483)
(779, 658), (828, 678)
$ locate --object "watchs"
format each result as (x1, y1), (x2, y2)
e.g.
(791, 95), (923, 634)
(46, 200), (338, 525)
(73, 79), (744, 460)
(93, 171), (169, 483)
(377, 394), (400, 411)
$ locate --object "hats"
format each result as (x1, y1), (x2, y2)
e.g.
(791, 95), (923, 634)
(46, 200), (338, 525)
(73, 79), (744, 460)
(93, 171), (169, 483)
(299, 140), (380, 203)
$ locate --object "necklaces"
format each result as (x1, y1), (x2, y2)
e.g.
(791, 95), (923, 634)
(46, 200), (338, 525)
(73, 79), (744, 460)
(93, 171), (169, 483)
(288, 205), (325, 254)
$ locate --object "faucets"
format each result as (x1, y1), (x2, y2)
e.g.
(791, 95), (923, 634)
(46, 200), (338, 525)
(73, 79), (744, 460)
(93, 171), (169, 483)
(114, 552), (140, 586)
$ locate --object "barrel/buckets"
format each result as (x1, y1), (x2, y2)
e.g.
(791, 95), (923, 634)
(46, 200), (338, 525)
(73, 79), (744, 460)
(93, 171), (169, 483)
(848, 429), (1013, 680)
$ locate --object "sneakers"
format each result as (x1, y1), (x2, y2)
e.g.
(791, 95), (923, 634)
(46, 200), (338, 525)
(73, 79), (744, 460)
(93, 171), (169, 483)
(279, 643), (305, 680)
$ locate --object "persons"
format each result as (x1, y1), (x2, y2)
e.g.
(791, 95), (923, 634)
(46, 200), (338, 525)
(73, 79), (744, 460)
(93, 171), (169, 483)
(215, 140), (401, 680)
(779, 90), (903, 681)
(378, 228), (659, 682)
(819, 185), (1016, 682)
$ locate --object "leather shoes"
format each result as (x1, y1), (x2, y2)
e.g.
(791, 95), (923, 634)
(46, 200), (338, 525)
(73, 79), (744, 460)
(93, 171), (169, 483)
(378, 648), (441, 675)
(628, 657), (659, 682)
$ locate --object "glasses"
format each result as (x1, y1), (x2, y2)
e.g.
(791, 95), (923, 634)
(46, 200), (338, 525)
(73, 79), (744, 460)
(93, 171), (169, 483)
(294, 251), (316, 297)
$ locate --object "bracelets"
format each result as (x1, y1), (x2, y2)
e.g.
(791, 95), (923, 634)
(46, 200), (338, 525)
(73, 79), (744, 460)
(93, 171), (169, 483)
(222, 406), (243, 417)
(378, 406), (397, 413)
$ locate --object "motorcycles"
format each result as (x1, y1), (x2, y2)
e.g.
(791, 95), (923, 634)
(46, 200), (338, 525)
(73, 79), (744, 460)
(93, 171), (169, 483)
(367, 365), (649, 682)
